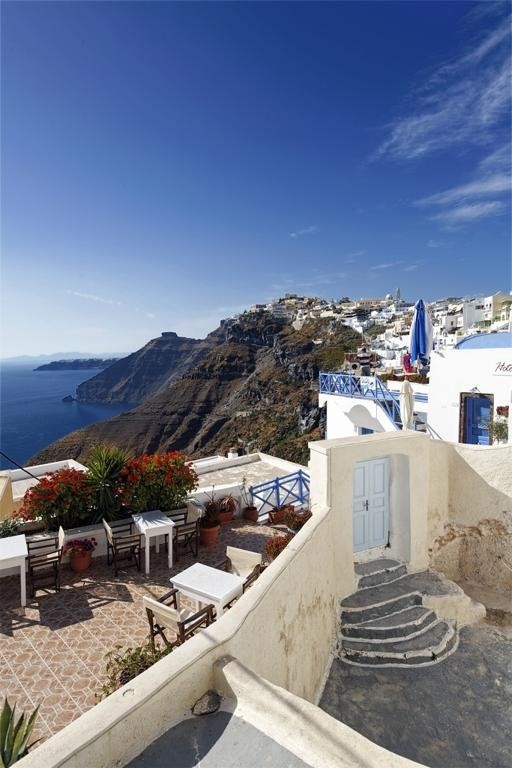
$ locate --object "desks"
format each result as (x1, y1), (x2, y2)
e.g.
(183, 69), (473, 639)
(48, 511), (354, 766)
(1, 535), (28, 607)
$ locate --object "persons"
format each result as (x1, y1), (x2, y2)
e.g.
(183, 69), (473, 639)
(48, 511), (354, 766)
(355, 346), (373, 376)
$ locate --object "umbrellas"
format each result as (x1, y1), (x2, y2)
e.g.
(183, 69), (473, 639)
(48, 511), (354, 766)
(407, 297), (435, 375)
(396, 376), (415, 431)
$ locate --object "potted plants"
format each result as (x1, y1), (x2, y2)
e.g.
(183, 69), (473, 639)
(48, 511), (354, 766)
(205, 477), (258, 523)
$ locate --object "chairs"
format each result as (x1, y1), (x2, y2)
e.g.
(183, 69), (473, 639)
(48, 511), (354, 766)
(25, 510), (269, 643)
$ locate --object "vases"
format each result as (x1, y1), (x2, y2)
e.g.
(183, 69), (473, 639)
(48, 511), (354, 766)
(270, 506), (311, 524)
